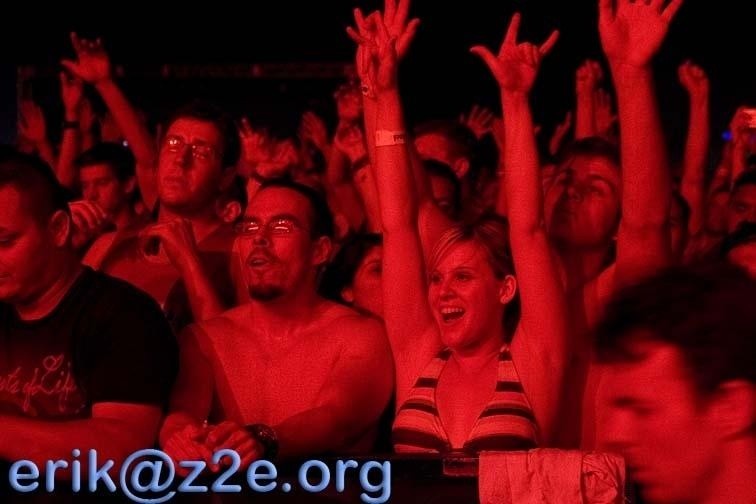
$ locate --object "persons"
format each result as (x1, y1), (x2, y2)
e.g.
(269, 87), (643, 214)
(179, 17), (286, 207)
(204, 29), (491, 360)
(584, 257), (756, 504)
(1, 0), (755, 478)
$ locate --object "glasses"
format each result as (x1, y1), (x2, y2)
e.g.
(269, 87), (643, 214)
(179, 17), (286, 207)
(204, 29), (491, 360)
(165, 135), (212, 161)
(243, 218), (294, 235)
